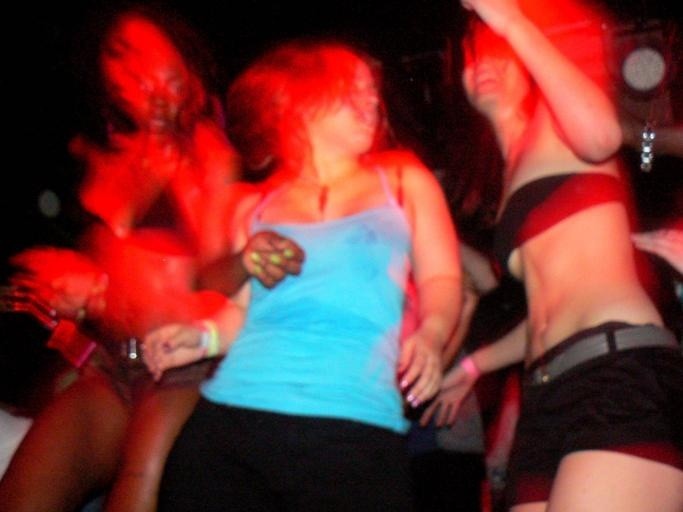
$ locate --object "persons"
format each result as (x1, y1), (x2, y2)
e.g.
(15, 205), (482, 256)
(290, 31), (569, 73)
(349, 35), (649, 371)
(0, 1), (683, 512)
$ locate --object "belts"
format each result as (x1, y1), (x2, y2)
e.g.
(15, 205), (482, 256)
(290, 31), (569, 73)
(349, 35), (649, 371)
(522, 326), (679, 389)
